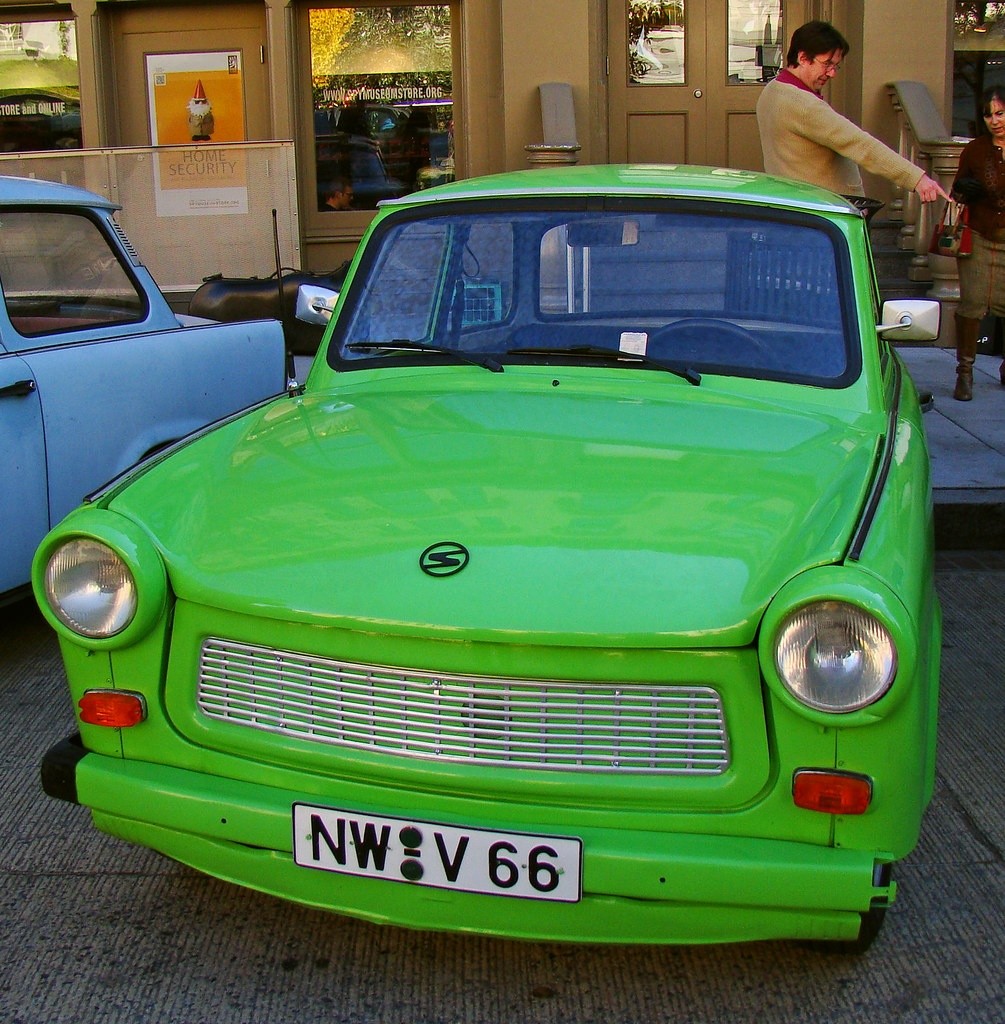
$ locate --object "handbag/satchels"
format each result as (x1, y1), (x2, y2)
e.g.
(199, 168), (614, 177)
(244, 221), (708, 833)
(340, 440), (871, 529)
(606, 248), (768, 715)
(927, 194), (972, 258)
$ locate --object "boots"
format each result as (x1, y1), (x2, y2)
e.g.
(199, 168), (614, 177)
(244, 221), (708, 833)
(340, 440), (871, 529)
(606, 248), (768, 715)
(952, 313), (980, 401)
(999, 359), (1005, 388)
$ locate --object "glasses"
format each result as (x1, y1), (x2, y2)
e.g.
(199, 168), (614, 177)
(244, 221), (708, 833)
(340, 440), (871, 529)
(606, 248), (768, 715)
(343, 191), (353, 197)
(811, 55), (841, 72)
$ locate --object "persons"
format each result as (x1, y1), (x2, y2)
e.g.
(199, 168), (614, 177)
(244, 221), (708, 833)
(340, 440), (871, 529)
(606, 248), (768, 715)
(436, 118), (454, 159)
(757, 20), (951, 219)
(945, 85), (1005, 401)
(318, 177), (356, 211)
(317, 144), (352, 177)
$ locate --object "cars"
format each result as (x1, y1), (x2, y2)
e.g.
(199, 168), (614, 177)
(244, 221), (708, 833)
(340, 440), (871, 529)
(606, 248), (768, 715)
(314, 134), (398, 209)
(316, 105), (441, 174)
(18, 163), (946, 952)
(1, 176), (289, 595)
